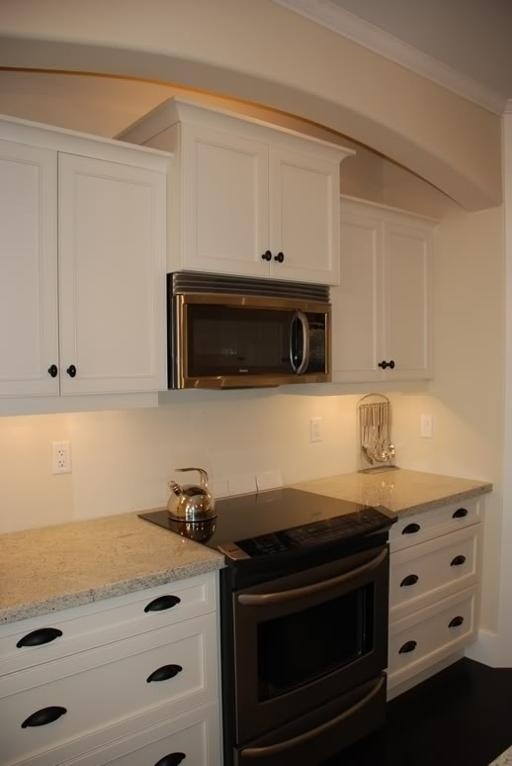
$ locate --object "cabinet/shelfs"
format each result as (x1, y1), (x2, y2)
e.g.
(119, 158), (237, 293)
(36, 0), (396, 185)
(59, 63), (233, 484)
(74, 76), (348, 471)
(388, 496), (484, 703)
(0, 113), (172, 412)
(337, 196), (437, 391)
(115, 97), (357, 282)
(0, 574), (222, 766)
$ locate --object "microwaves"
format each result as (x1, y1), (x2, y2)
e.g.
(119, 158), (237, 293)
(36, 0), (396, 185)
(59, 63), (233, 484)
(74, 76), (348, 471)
(168, 272), (333, 391)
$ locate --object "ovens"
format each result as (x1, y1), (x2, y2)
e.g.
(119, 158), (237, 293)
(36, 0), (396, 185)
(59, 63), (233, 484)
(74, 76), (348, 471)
(218, 533), (389, 766)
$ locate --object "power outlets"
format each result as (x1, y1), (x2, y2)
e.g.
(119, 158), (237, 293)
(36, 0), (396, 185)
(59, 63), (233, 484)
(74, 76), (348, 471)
(52, 441), (70, 475)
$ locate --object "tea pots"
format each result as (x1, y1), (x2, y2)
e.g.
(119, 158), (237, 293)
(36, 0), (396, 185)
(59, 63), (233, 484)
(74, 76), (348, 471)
(167, 466), (218, 522)
